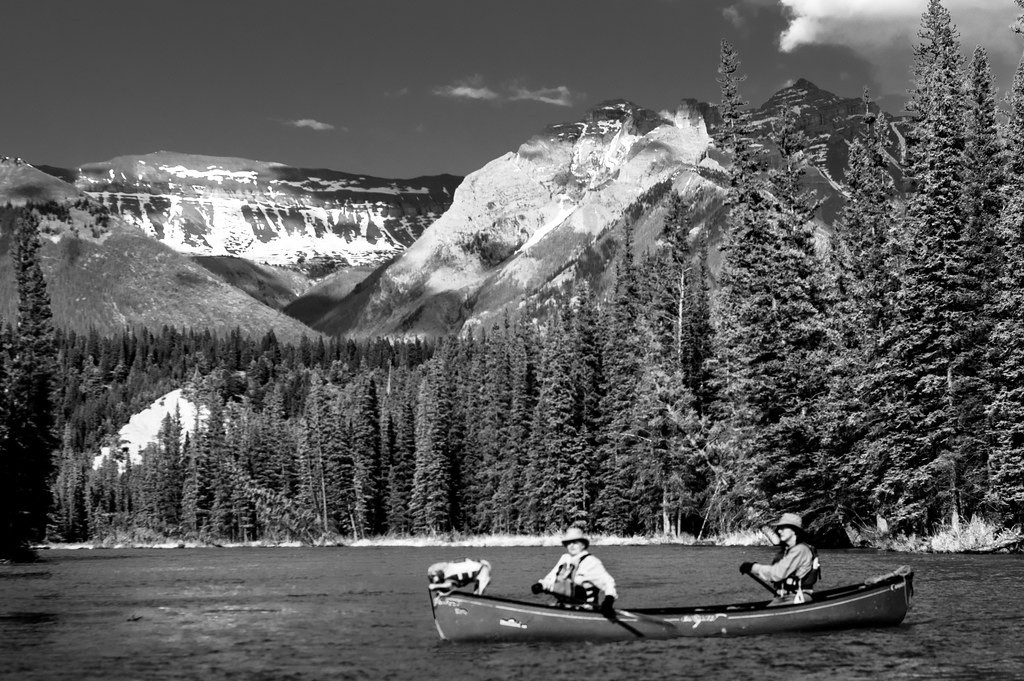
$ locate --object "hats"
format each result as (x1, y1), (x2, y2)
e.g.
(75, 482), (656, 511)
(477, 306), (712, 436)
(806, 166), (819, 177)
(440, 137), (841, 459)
(770, 513), (803, 528)
(561, 528), (590, 549)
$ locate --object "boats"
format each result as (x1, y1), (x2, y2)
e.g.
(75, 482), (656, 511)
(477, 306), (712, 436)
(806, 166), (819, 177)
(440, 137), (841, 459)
(428, 565), (916, 642)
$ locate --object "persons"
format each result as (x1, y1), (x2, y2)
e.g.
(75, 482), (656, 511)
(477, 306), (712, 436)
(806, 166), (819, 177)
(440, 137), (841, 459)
(534, 528), (618, 622)
(740, 513), (820, 609)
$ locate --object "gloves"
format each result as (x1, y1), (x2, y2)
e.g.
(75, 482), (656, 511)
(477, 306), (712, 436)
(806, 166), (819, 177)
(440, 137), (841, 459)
(531, 583), (542, 594)
(601, 595), (614, 610)
(739, 561), (757, 575)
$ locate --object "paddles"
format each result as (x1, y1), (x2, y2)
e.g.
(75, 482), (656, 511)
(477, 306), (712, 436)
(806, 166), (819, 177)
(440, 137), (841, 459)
(543, 590), (675, 628)
(746, 572), (778, 597)
